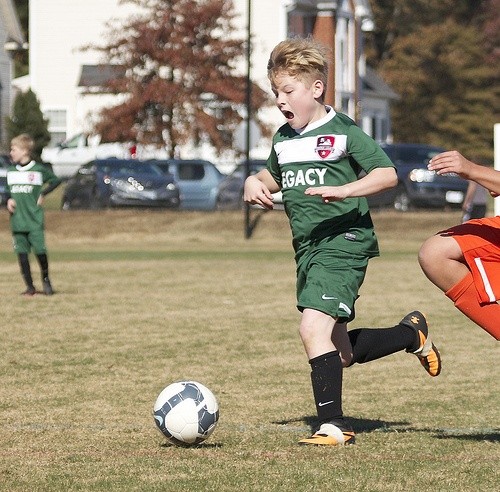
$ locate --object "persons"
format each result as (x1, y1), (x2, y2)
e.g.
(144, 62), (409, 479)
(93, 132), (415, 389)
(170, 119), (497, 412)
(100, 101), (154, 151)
(418, 151), (500, 342)
(4, 134), (61, 296)
(244, 38), (442, 446)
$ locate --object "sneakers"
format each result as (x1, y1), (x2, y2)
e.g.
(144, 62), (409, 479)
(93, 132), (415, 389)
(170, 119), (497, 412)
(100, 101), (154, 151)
(399, 311), (442, 377)
(297, 421), (356, 447)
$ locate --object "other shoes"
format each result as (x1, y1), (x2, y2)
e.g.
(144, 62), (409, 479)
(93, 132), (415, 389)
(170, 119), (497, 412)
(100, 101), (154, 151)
(22, 284), (35, 296)
(42, 281), (52, 295)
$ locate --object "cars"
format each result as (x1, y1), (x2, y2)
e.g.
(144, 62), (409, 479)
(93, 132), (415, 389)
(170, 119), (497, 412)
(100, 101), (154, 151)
(358, 142), (471, 214)
(140, 158), (233, 213)
(59, 158), (182, 212)
(213, 157), (286, 212)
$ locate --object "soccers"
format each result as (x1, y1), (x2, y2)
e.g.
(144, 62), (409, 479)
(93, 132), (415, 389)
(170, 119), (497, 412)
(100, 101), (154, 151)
(153, 379), (219, 446)
